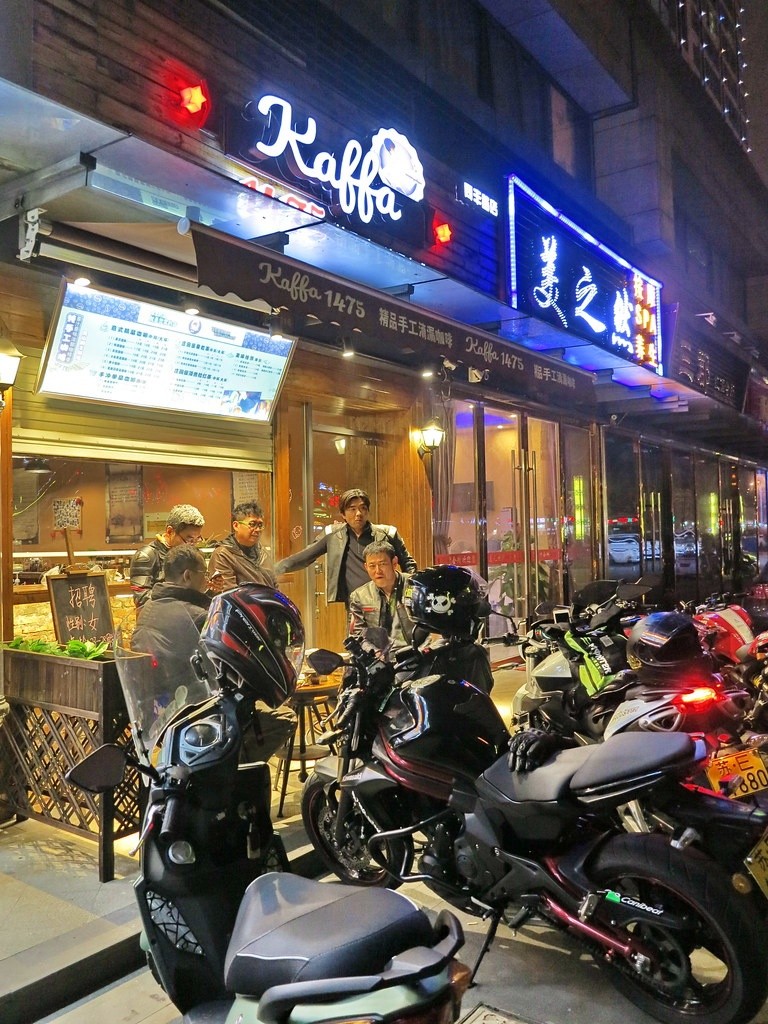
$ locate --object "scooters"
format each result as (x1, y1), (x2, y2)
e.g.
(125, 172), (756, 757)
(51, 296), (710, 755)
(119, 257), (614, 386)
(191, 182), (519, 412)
(66, 582), (473, 1024)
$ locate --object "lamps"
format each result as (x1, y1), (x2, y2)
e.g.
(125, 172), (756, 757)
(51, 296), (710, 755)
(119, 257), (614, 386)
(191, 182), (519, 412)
(183, 299), (200, 316)
(422, 364), (434, 378)
(334, 434), (346, 454)
(269, 317), (284, 342)
(71, 271), (90, 287)
(25, 460), (51, 474)
(342, 338), (354, 357)
(413, 416), (445, 459)
(694, 311), (760, 359)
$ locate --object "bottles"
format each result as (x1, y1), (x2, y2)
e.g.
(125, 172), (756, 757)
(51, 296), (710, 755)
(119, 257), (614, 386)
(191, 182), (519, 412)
(118, 558), (124, 578)
(96, 556), (115, 569)
(123, 559), (131, 580)
(24, 558), (48, 572)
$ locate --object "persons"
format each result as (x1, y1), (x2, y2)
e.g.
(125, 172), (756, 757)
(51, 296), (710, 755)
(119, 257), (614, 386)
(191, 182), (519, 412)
(505, 524), (519, 537)
(278, 488), (418, 611)
(351, 542), (441, 669)
(643, 504), (659, 539)
(209, 502), (280, 590)
(130, 504), (223, 622)
(131, 544), (298, 762)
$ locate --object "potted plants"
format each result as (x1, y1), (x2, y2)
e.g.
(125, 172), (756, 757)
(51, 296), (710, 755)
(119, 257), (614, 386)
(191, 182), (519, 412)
(0, 639), (153, 720)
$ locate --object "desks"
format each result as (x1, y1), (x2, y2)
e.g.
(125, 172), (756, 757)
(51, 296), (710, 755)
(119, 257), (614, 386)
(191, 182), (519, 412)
(273, 679), (344, 817)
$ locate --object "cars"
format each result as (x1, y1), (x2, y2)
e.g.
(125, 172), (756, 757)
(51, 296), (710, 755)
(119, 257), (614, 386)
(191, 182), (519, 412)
(607, 528), (703, 564)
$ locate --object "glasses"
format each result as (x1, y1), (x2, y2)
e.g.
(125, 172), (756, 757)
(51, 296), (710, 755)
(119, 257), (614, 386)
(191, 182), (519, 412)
(239, 521), (265, 530)
(180, 568), (210, 580)
(177, 532), (203, 544)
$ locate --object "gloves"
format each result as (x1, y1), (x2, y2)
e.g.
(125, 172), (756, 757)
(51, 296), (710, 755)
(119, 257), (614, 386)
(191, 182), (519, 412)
(505, 728), (558, 774)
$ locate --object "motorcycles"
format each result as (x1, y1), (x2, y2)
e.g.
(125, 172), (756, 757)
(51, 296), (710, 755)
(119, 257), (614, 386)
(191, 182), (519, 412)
(298, 563), (767, 1024)
(476, 567), (766, 747)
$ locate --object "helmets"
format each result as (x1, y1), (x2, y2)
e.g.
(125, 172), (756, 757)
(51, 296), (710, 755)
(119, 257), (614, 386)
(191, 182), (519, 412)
(626, 612), (698, 687)
(408, 564), (489, 638)
(198, 582), (305, 709)
(747, 630), (768, 658)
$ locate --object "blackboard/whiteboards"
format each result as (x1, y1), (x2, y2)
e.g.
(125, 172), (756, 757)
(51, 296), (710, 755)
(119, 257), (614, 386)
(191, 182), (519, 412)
(45, 570), (117, 650)
